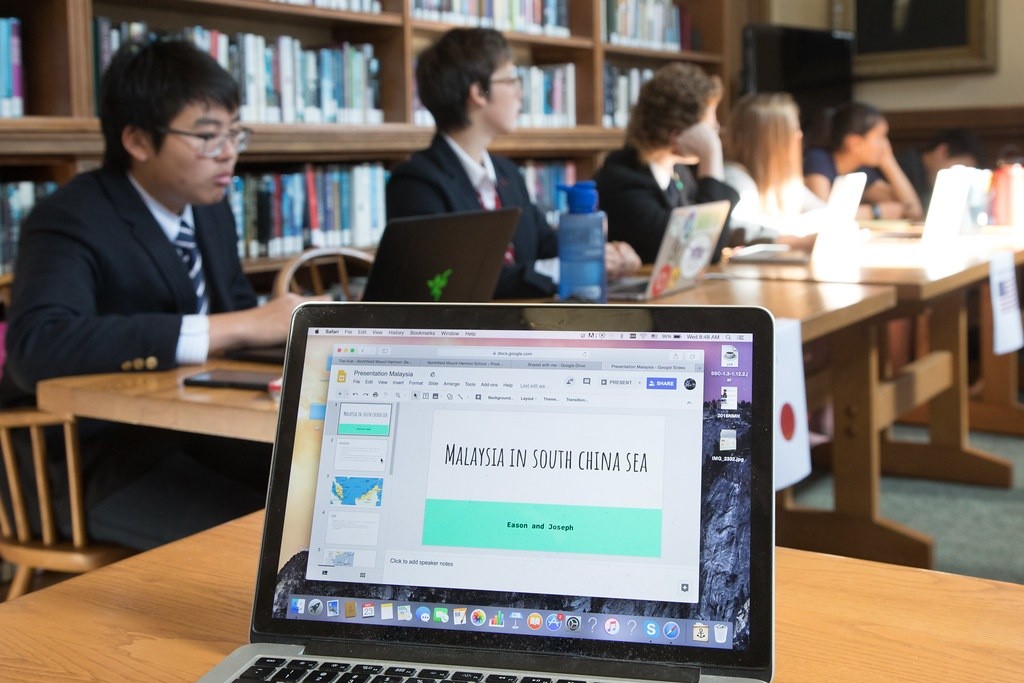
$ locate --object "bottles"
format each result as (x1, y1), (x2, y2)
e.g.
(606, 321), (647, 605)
(556, 180), (607, 303)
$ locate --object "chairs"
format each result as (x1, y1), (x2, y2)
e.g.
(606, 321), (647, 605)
(274, 247), (379, 306)
(0, 275), (132, 601)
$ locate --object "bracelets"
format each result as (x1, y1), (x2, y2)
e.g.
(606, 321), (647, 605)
(871, 204), (882, 221)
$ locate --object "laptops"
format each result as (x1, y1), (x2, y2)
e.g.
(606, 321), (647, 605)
(193, 166), (993, 683)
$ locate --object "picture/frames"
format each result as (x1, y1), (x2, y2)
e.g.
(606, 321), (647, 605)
(828, 0), (998, 83)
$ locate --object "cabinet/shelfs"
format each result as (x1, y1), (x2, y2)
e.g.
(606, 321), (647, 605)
(0, 0), (771, 299)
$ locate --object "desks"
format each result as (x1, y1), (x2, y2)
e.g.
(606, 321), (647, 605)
(0, 217), (1024, 683)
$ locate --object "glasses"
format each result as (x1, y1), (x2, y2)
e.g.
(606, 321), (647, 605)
(169, 128), (250, 158)
(494, 76), (526, 88)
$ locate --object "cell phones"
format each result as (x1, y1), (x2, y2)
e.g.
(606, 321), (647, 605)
(183, 368), (283, 391)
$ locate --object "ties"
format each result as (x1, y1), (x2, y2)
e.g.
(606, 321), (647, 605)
(473, 163), (515, 263)
(667, 178), (679, 208)
(174, 221), (209, 315)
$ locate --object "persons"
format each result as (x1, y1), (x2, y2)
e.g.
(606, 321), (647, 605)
(895, 128), (985, 211)
(0, 37), (336, 548)
(595, 59), (741, 266)
(804, 101), (923, 221)
(385, 24), (641, 330)
(722, 89), (831, 252)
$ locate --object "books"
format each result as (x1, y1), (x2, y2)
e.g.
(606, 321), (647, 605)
(94, 16), (385, 123)
(603, 0), (681, 52)
(413, 62), (577, 131)
(275, 0), (385, 13)
(605, 66), (657, 130)
(2, 181), (58, 273)
(411, 0), (573, 38)
(515, 160), (576, 230)
(223, 162), (391, 261)
(1, 16), (24, 118)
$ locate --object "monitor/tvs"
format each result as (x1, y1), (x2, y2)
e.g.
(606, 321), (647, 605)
(742, 23), (854, 133)
